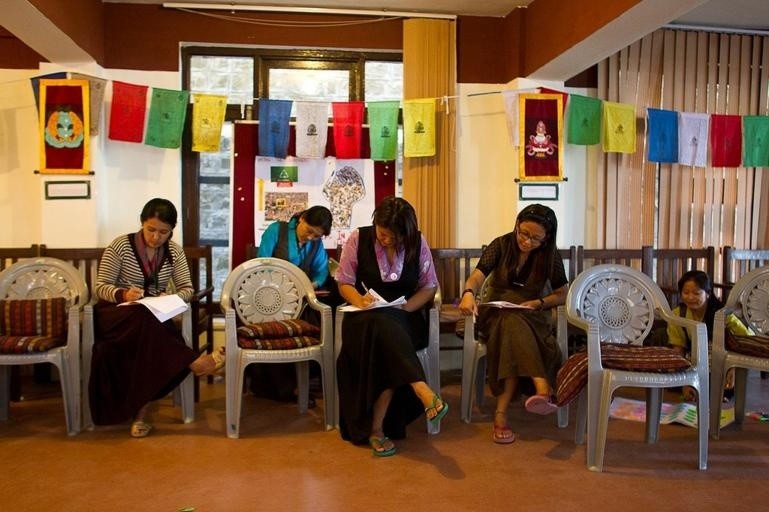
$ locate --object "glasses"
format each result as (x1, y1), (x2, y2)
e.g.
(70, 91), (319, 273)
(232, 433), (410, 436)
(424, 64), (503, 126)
(518, 223), (544, 243)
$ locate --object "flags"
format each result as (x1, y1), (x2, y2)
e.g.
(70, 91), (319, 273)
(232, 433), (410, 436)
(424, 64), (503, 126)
(497, 86), (768, 168)
(332, 98), (436, 161)
(258, 98), (328, 159)
(109, 81), (228, 154)
(31, 71), (106, 137)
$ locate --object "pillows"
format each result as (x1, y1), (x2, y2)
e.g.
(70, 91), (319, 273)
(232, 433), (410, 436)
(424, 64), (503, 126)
(0, 298), (67, 354)
(236, 319), (321, 350)
(727, 335), (769, 359)
(556, 342), (691, 407)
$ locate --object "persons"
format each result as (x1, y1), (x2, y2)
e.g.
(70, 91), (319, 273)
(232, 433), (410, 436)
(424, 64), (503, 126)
(665, 270), (754, 409)
(334, 197), (450, 458)
(87, 197), (226, 438)
(458, 203), (570, 445)
(249, 205), (333, 410)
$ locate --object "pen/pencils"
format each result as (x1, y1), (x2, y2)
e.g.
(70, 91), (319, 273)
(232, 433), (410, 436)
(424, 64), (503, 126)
(361, 280), (376, 302)
(472, 312), (476, 324)
(127, 280), (145, 298)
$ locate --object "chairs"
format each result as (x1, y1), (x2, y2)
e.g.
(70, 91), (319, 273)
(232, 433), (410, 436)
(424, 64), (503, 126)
(711, 266), (769, 441)
(459, 258), (569, 429)
(0, 244), (39, 401)
(220, 257), (334, 439)
(555, 263), (711, 473)
(334, 303), (442, 435)
(82, 259), (195, 431)
(0, 258), (91, 436)
(39, 244), (214, 402)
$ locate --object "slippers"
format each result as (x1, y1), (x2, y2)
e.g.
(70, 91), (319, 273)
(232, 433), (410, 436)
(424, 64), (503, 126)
(494, 424), (515, 443)
(425, 392), (449, 424)
(526, 395), (558, 415)
(370, 436), (396, 456)
(131, 421), (151, 436)
(196, 345), (226, 376)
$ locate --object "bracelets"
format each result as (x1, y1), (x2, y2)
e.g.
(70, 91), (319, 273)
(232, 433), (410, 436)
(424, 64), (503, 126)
(461, 288), (475, 299)
(537, 298), (545, 312)
